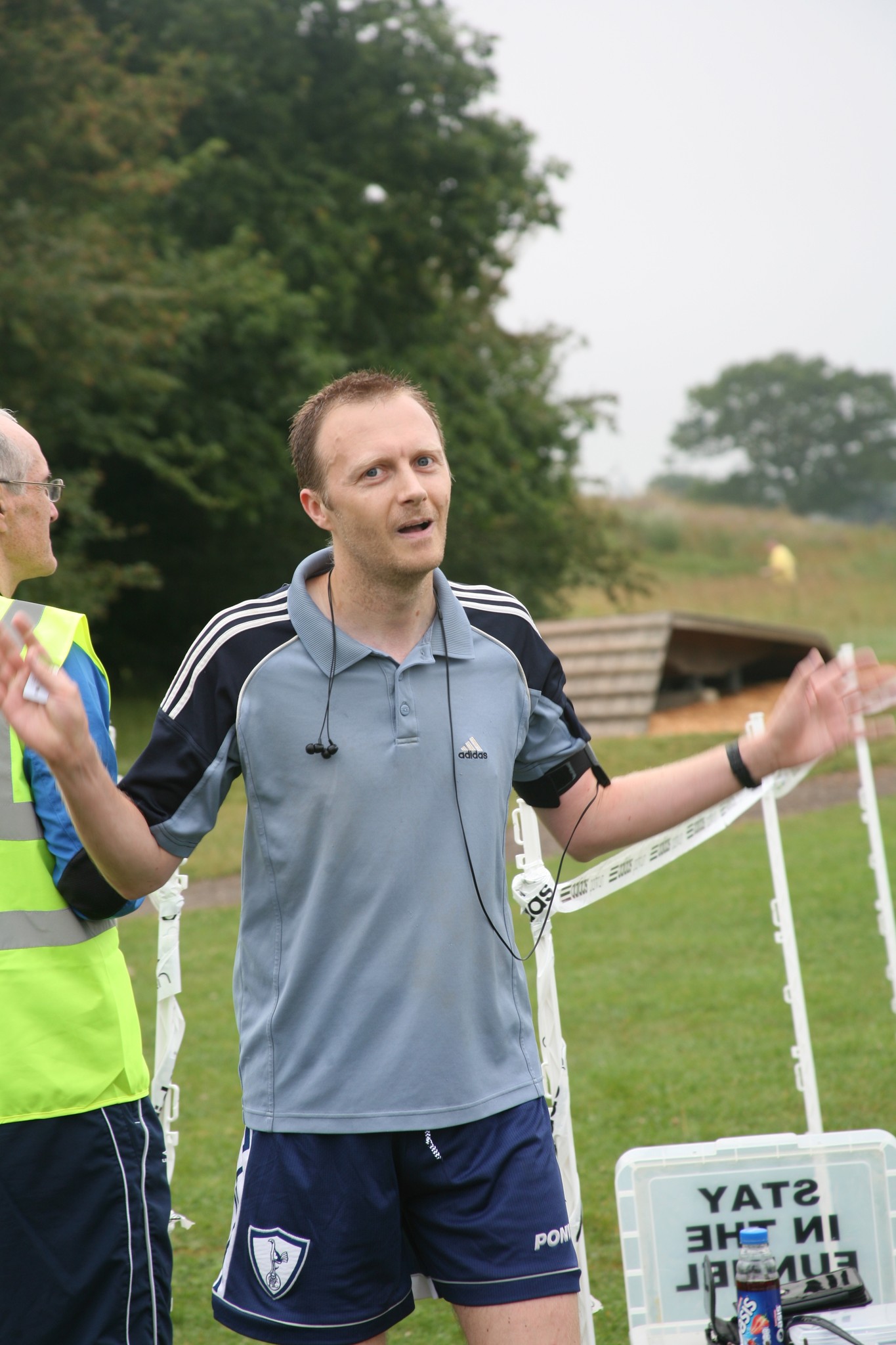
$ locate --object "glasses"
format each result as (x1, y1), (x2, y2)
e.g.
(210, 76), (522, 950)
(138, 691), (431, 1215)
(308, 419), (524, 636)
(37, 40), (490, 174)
(0, 478), (66, 502)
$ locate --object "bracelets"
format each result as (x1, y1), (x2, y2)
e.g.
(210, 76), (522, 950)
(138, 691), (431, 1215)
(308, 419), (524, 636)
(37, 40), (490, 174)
(725, 737), (761, 790)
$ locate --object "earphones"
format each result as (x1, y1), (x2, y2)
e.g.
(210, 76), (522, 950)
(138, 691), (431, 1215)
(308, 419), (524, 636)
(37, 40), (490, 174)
(320, 744), (338, 759)
(305, 743), (324, 755)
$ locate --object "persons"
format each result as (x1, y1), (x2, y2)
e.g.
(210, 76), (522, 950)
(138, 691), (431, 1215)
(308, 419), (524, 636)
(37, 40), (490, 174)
(1, 369), (896, 1345)
(0, 406), (175, 1345)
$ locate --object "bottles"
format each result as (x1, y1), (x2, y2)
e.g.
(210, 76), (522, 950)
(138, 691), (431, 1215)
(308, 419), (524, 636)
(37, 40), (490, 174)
(735, 1227), (785, 1345)
